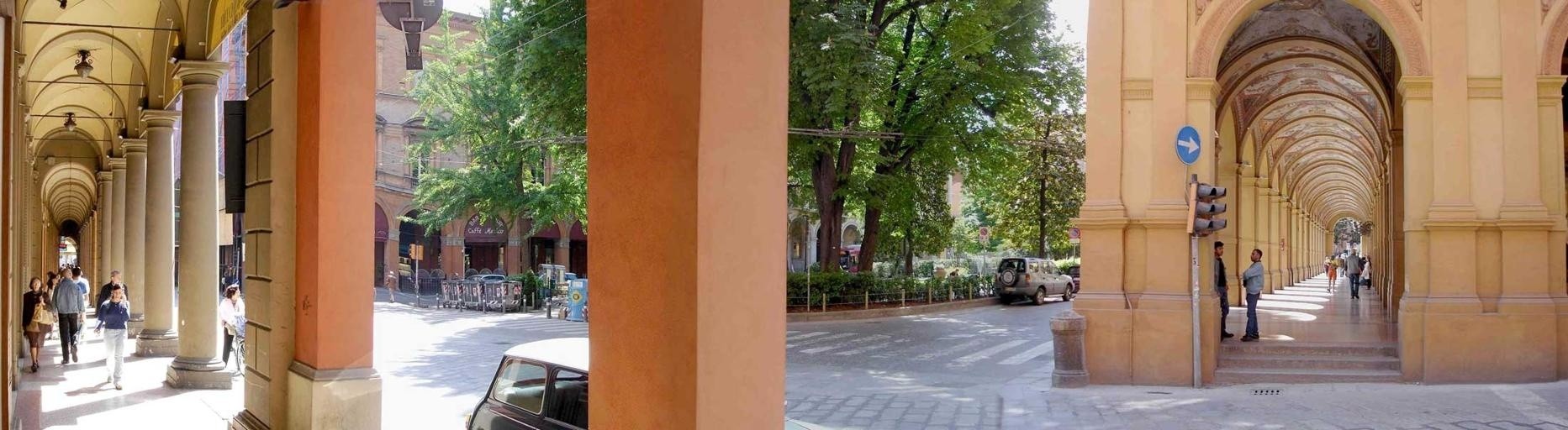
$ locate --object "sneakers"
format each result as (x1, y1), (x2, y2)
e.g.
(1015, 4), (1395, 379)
(1327, 289), (1335, 294)
(61, 350), (78, 364)
(1350, 294), (1359, 299)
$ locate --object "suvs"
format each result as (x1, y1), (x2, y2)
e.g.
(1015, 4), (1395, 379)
(991, 257), (1074, 306)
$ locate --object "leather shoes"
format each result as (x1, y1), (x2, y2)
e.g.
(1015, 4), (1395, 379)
(1221, 329), (1234, 342)
(1241, 334), (1259, 342)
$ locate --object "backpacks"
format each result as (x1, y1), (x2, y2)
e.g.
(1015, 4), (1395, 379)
(32, 305), (54, 325)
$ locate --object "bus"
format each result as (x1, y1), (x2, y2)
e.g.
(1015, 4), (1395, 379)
(839, 245), (861, 273)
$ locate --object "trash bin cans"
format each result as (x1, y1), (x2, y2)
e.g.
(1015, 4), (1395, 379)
(440, 280), (522, 313)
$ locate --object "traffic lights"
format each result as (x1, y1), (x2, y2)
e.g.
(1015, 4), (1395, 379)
(408, 244), (415, 259)
(1185, 181), (1227, 236)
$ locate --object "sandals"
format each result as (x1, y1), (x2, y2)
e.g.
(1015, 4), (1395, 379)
(31, 363), (40, 373)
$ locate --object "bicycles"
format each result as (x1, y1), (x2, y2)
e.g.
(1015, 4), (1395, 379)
(229, 329), (245, 376)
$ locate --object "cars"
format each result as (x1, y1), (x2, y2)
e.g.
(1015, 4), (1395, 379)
(1066, 266), (1079, 294)
(465, 337), (809, 430)
(534, 272), (578, 283)
(463, 273), (506, 282)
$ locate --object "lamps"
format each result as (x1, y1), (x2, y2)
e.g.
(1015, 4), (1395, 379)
(63, 113), (78, 133)
(74, 47), (94, 80)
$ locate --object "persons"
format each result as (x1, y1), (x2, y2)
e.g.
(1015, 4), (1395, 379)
(218, 284), (244, 366)
(950, 268), (960, 278)
(1240, 249), (1264, 342)
(1322, 248), (1373, 300)
(22, 261), (131, 390)
(384, 270), (399, 303)
(1213, 241), (1234, 342)
